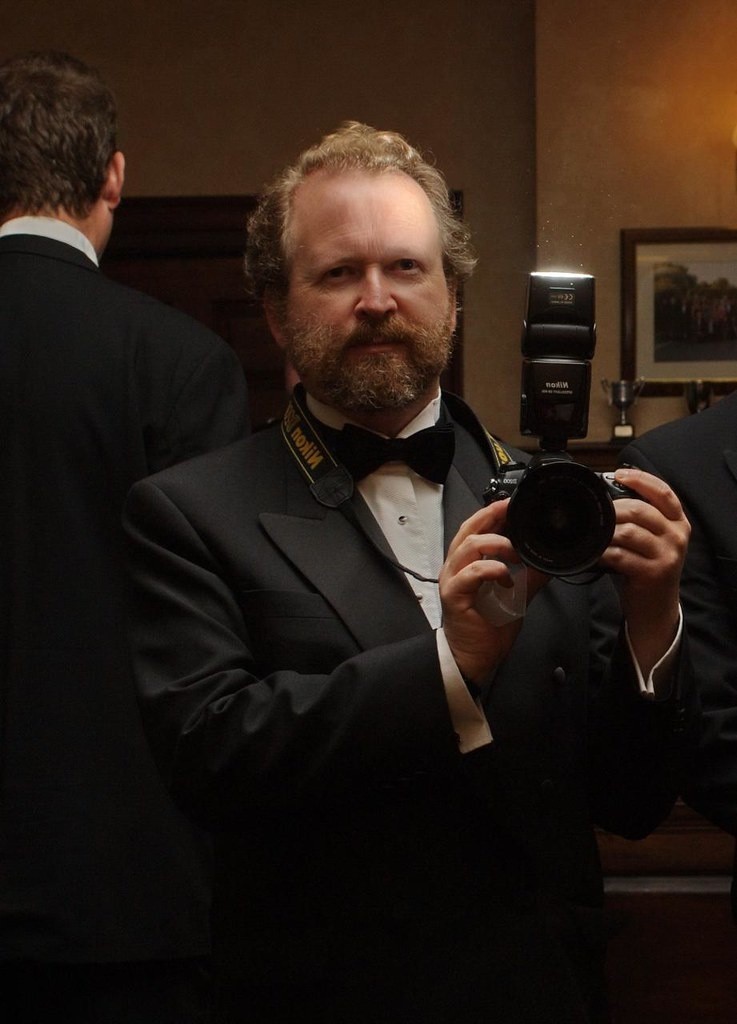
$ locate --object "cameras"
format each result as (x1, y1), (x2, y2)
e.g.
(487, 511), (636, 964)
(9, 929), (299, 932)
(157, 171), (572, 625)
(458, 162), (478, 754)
(484, 270), (650, 580)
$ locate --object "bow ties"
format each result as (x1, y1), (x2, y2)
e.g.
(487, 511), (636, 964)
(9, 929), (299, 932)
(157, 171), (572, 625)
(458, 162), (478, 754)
(336, 422), (455, 484)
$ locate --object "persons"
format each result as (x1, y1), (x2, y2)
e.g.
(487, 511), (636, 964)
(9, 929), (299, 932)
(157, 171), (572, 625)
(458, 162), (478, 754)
(616, 390), (737, 923)
(1, 51), (250, 1024)
(123, 125), (696, 1023)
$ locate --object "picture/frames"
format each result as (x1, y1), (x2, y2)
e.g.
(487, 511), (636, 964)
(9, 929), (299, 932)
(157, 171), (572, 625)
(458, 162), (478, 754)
(618, 225), (737, 400)
